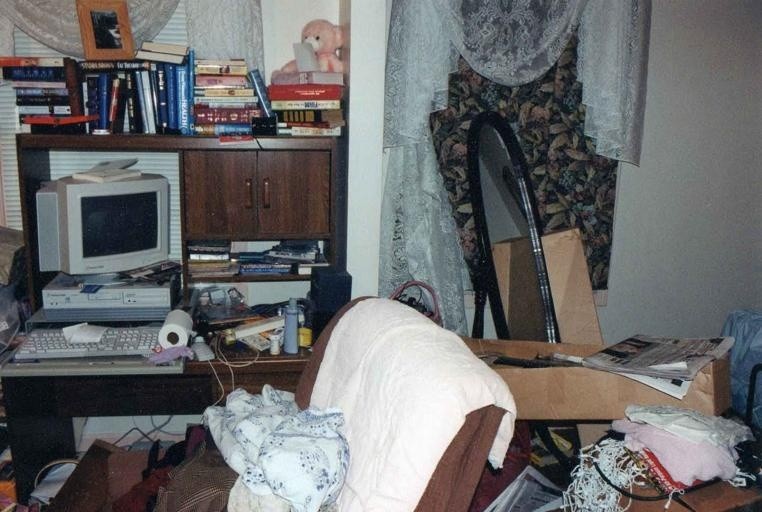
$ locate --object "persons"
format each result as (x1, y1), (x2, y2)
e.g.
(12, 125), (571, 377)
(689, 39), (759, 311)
(228, 288), (249, 312)
(197, 291), (210, 313)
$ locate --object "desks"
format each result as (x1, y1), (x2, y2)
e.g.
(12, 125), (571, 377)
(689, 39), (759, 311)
(3, 341), (311, 510)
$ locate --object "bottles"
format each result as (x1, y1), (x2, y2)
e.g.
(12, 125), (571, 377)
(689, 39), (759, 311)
(284, 298), (300, 353)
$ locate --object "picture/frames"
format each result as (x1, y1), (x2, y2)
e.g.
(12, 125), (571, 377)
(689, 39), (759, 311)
(76, 0), (134, 61)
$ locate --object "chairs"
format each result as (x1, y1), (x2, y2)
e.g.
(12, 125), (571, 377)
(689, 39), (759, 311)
(103, 295), (517, 510)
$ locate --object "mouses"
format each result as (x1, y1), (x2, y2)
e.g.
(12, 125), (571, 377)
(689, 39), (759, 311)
(192, 342), (215, 361)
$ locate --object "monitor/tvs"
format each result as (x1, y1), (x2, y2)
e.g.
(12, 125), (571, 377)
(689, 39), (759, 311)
(35, 171), (169, 286)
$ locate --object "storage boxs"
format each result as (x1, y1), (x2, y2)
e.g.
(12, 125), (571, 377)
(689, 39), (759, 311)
(460, 334), (731, 421)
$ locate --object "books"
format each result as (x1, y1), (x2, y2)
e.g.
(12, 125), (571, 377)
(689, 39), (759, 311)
(187, 239), (331, 277)
(530, 431), (574, 467)
(72, 158), (142, 184)
(0, 41), (345, 136)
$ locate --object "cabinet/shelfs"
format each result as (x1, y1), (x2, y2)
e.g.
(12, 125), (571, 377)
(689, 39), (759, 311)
(183, 147), (331, 240)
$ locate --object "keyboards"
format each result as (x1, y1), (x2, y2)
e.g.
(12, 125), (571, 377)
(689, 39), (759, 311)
(13, 325), (161, 360)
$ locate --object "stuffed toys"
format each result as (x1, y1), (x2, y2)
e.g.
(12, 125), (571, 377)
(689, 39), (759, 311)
(281, 19), (345, 72)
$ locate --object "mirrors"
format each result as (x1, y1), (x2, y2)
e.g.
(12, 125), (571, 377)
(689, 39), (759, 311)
(466, 110), (560, 344)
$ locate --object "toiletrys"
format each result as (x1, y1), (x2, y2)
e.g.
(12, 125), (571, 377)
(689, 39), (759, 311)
(283, 297), (298, 354)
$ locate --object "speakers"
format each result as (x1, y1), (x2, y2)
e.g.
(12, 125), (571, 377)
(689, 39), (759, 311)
(310, 266), (351, 345)
